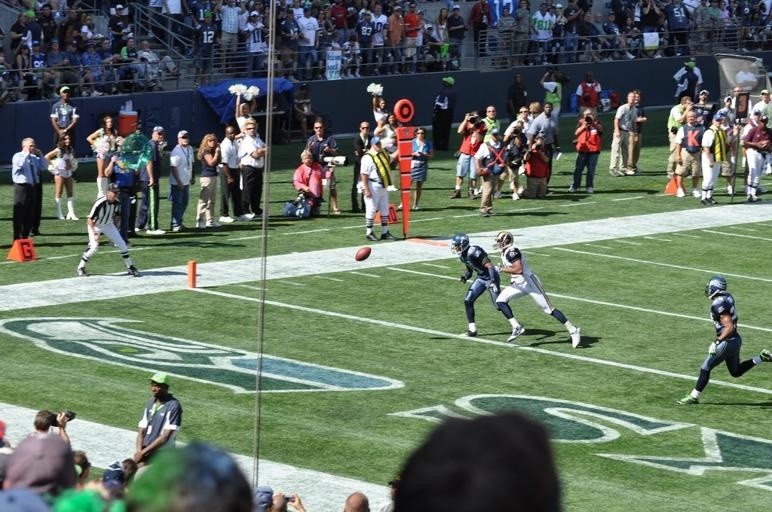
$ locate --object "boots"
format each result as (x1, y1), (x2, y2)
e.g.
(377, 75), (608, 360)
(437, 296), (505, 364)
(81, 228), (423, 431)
(55, 197), (65, 220)
(66, 197), (79, 221)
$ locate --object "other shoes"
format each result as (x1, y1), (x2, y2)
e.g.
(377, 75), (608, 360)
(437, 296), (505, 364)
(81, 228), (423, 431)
(567, 184), (580, 191)
(609, 167), (638, 176)
(667, 174), (759, 207)
(587, 186), (593, 193)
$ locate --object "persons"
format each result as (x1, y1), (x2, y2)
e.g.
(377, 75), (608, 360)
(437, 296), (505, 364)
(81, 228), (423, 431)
(129, 439), (369, 512)
(432, 76), (456, 151)
(0, 411), (137, 511)
(451, 233), (503, 337)
(134, 371), (182, 467)
(393, 411), (562, 511)
(273, 0), (468, 82)
(11, 86), (269, 276)
(1, 1), (273, 101)
(492, 231), (581, 348)
(450, 2), (770, 217)
(675, 275), (772, 404)
(293, 83), (432, 241)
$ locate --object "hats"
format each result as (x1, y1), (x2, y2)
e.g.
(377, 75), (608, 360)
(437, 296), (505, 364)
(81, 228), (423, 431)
(60, 86), (69, 93)
(152, 126), (166, 133)
(685, 62), (694, 69)
(148, 373), (169, 385)
(177, 130), (189, 138)
(442, 76), (454, 84)
(6, 432), (77, 492)
(370, 136), (380, 145)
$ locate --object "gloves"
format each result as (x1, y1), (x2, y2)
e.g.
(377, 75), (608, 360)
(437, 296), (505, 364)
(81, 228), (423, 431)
(709, 342), (717, 357)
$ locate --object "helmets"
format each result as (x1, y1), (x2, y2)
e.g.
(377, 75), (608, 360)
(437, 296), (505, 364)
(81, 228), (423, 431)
(705, 276), (726, 299)
(451, 234), (469, 254)
(492, 231), (513, 250)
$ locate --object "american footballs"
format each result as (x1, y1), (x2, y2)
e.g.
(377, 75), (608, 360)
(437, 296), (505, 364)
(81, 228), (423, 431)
(355, 247), (371, 261)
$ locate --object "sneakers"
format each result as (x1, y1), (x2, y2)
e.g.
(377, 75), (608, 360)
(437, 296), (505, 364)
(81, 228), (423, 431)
(56, 209), (255, 277)
(675, 395), (697, 405)
(759, 349), (772, 362)
(460, 327), (582, 348)
(366, 231), (378, 240)
(448, 183), (549, 217)
(381, 231), (395, 239)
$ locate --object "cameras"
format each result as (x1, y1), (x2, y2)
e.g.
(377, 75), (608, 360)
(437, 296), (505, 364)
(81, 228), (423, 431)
(467, 113), (480, 126)
(49, 408), (76, 425)
(534, 139), (546, 153)
(512, 124), (522, 137)
(582, 113), (593, 127)
(322, 154), (350, 168)
(283, 495), (294, 504)
